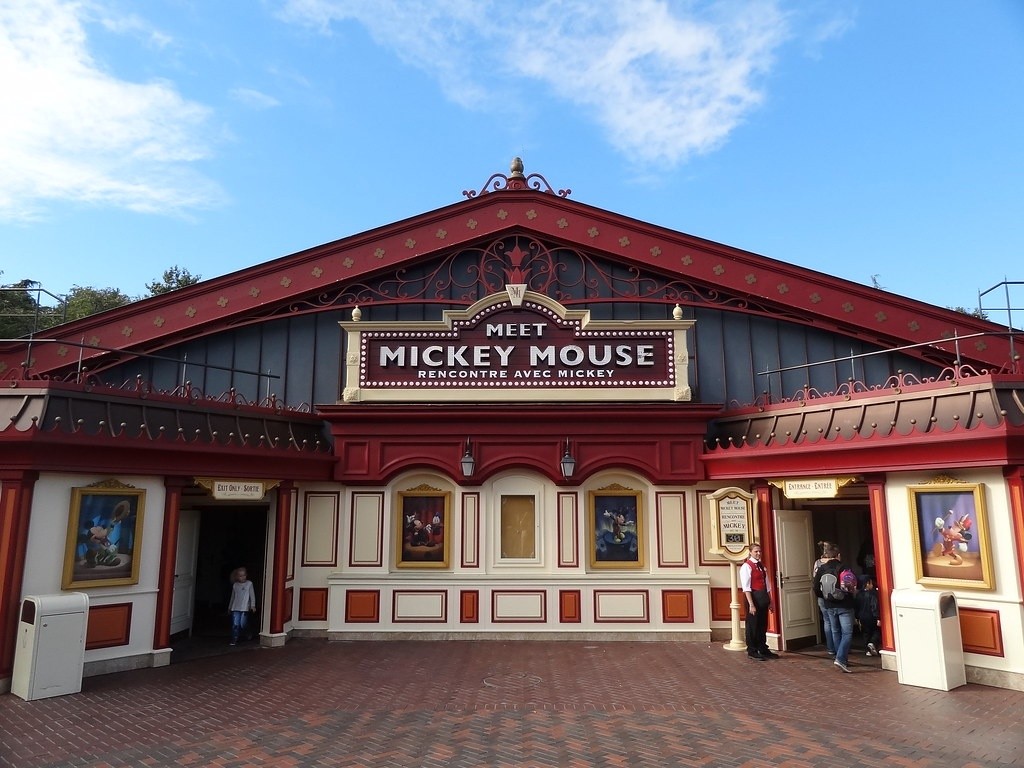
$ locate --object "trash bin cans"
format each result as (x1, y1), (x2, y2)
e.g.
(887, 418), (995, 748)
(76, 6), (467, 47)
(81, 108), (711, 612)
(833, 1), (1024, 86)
(890, 587), (968, 691)
(10, 590), (91, 702)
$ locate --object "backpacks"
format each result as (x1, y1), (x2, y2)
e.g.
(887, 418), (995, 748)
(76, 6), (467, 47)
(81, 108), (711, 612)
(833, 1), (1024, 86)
(839, 568), (857, 593)
(818, 563), (845, 603)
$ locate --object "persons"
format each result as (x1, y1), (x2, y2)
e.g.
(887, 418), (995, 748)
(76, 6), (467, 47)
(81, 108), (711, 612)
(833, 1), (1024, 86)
(812, 535), (882, 673)
(739, 542), (779, 662)
(855, 574), (882, 656)
(227, 567), (256, 646)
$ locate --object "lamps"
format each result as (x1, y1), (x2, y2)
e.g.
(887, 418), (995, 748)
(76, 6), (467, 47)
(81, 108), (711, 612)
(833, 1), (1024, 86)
(560, 437), (575, 478)
(461, 437), (476, 476)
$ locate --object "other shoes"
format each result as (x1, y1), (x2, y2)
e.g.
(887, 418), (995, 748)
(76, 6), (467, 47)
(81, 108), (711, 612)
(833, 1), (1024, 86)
(748, 648), (765, 661)
(229, 638), (239, 646)
(834, 659), (852, 673)
(248, 631), (253, 640)
(758, 647), (780, 659)
(865, 642), (878, 657)
(827, 650), (836, 655)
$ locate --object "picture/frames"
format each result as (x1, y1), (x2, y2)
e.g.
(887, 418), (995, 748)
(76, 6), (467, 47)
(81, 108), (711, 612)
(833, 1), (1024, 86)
(589, 483), (644, 568)
(61, 478), (147, 590)
(396, 483), (450, 568)
(905, 473), (996, 592)
